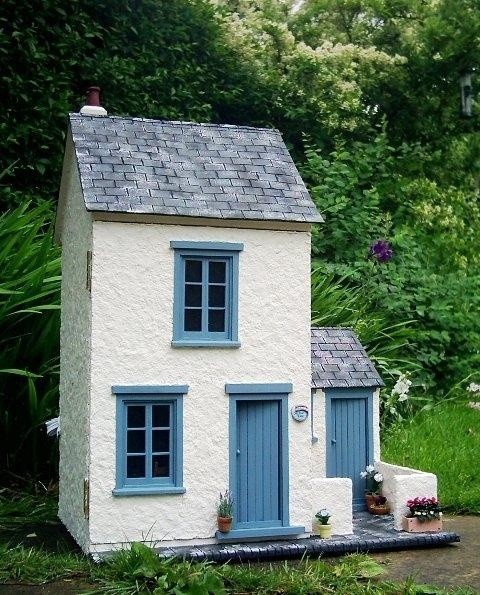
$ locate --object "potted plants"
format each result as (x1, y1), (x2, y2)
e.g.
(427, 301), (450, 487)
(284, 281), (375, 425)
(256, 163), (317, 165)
(217, 490), (234, 532)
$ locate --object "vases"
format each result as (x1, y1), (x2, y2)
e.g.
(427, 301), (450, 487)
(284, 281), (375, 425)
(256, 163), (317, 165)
(318, 525), (331, 539)
(370, 507), (390, 514)
(365, 493), (380, 512)
(402, 514), (442, 532)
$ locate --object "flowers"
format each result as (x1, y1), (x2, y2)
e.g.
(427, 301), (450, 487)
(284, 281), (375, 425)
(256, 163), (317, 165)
(360, 465), (384, 495)
(372, 495), (388, 508)
(315, 508), (332, 524)
(406, 496), (443, 521)
